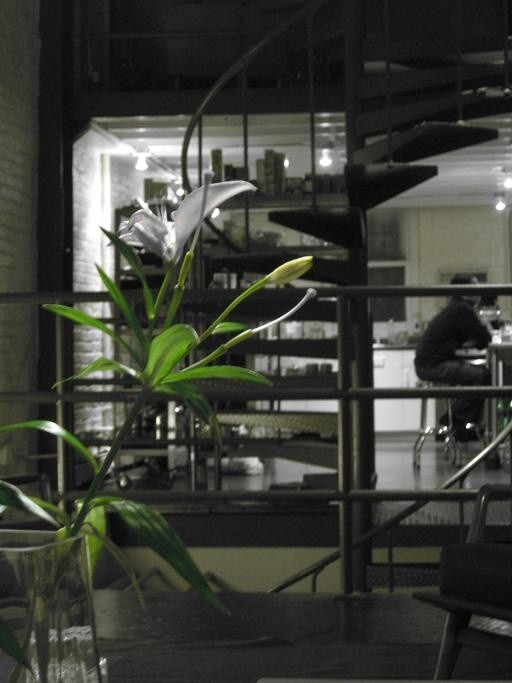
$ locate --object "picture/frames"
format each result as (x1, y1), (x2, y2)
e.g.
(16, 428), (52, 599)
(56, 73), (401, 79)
(1, 527), (108, 683)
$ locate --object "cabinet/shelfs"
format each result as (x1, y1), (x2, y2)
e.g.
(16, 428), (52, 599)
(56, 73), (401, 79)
(110, 205), (360, 489)
(253, 349), (439, 433)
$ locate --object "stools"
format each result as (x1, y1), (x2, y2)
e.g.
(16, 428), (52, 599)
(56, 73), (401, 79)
(412, 383), (461, 467)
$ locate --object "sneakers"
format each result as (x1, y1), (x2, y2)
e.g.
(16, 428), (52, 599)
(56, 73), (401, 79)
(439, 417), (470, 444)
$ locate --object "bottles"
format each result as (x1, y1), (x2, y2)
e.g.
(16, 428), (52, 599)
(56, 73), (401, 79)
(497, 397), (510, 438)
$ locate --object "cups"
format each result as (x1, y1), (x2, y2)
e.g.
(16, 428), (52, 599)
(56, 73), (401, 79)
(286, 363), (333, 377)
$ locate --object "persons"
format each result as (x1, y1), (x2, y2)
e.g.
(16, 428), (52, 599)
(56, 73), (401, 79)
(413, 271), (495, 442)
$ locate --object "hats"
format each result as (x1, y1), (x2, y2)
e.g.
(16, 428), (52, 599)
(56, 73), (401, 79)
(449, 273), (475, 297)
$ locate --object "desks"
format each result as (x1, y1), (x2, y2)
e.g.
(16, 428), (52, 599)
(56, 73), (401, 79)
(484, 342), (512, 471)
(35, 489), (511, 597)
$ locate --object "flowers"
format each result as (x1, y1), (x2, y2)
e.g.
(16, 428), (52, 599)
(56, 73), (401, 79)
(0, 168), (315, 630)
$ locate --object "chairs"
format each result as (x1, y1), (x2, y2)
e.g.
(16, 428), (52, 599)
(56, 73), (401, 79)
(412, 479), (509, 680)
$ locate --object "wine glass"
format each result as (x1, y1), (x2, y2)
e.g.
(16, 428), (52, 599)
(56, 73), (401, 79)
(487, 309), (507, 338)
